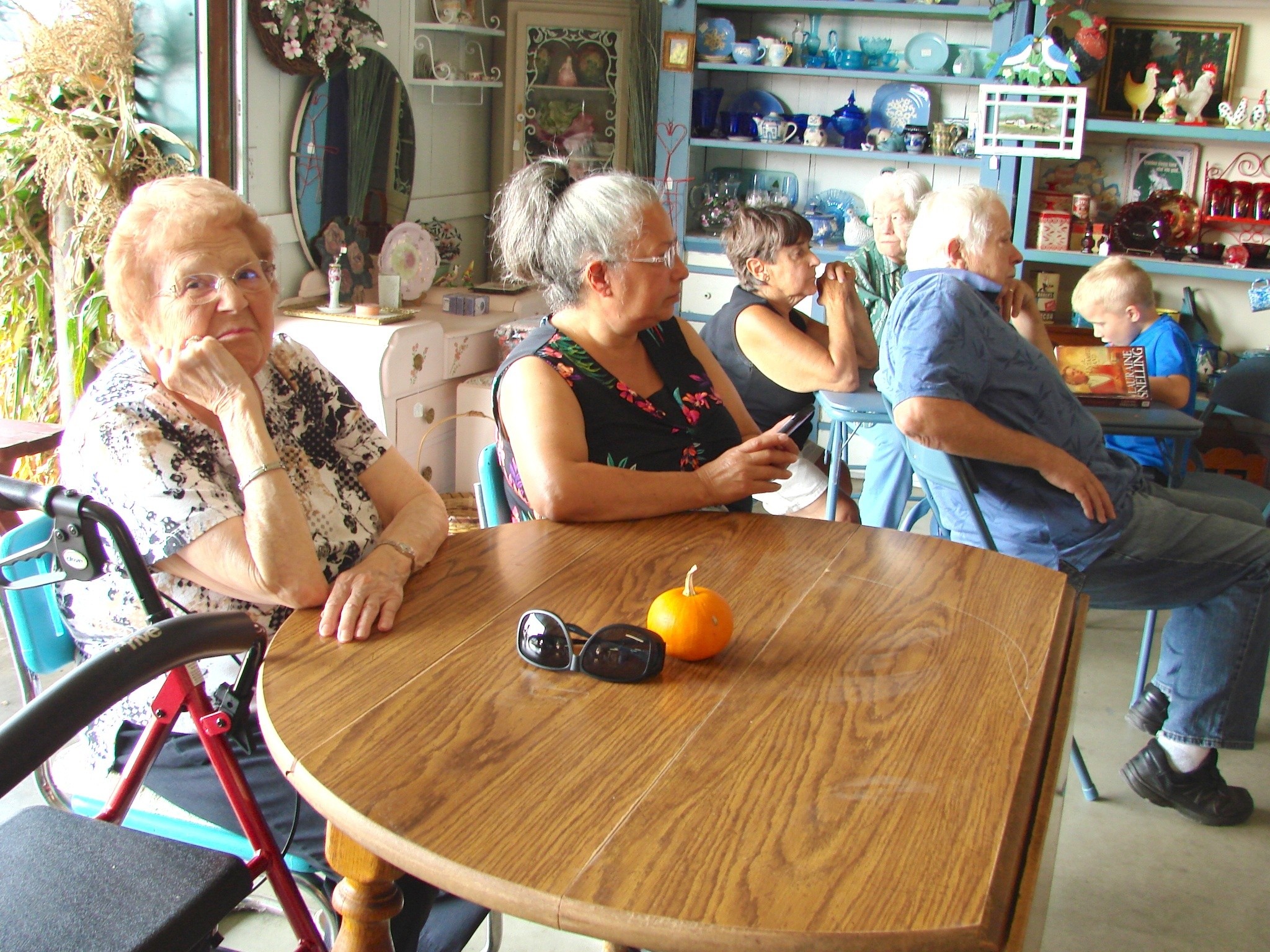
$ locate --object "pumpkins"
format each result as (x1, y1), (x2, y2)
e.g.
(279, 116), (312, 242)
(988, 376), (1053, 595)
(647, 564), (733, 661)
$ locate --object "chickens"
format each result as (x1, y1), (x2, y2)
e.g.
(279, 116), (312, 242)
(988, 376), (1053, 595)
(1173, 61), (1220, 124)
(1153, 69), (1185, 119)
(1218, 95), (1249, 126)
(1249, 89), (1269, 129)
(1123, 61), (1160, 123)
(1050, 13), (1110, 105)
(842, 200), (875, 248)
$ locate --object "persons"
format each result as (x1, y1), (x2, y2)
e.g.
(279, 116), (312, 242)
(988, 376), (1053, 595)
(879, 179), (1270, 829)
(54, 174), (492, 952)
(699, 205), (878, 525)
(673, 41), (686, 64)
(844, 169), (951, 540)
(558, 56), (577, 86)
(1148, 168), (1172, 200)
(492, 159), (801, 524)
(1073, 256), (1198, 487)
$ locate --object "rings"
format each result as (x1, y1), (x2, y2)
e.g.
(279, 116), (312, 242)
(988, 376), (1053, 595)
(844, 270), (851, 274)
(184, 336), (202, 347)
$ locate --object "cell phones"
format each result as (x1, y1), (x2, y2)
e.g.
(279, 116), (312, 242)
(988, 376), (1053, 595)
(778, 403), (815, 436)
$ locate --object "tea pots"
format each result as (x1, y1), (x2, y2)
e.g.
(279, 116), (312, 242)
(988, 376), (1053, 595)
(752, 111), (797, 146)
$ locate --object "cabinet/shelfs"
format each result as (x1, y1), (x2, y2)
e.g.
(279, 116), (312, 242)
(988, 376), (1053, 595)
(401, 0), (506, 106)
(1013, 0), (1270, 280)
(272, 288), (552, 497)
(385, 77), (414, 226)
(489, 0), (640, 210)
(652, 0), (1031, 340)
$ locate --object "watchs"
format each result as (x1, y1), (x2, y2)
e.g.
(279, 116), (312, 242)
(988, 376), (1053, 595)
(373, 539), (415, 576)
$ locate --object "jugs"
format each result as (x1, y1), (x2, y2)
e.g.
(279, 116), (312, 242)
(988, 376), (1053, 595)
(928, 121), (965, 156)
(689, 170), (741, 239)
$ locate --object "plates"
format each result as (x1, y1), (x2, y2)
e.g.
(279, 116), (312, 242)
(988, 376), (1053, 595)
(696, 16), (736, 57)
(414, 0), (468, 74)
(377, 222), (439, 302)
(728, 89), (784, 121)
(1112, 200), (1169, 254)
(802, 189), (867, 244)
(869, 81), (930, 131)
(1146, 188), (1203, 247)
(904, 31), (949, 71)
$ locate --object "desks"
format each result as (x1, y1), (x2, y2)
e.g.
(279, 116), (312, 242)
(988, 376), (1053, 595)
(256, 521), (1092, 952)
(811, 390), (1205, 704)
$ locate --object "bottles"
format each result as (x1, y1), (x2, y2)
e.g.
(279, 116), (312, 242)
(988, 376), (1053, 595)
(755, 10), (841, 70)
(953, 48), (974, 77)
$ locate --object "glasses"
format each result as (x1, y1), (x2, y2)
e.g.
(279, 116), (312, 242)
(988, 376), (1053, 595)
(516, 608), (666, 683)
(597, 238), (681, 269)
(145, 259), (275, 308)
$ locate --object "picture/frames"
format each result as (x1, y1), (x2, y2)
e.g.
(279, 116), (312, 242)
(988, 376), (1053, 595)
(660, 30), (696, 74)
(1121, 137), (1203, 204)
(1094, 14), (1244, 129)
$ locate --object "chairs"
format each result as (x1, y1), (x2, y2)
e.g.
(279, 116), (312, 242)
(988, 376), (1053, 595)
(471, 442), (511, 528)
(1180, 353), (1270, 510)
(880, 391), (1099, 804)
(0, 515), (503, 952)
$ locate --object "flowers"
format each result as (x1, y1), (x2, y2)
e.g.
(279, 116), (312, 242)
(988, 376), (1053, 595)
(257, 0), (388, 84)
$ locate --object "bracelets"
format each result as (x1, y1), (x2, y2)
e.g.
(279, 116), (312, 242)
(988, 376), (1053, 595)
(238, 458), (285, 490)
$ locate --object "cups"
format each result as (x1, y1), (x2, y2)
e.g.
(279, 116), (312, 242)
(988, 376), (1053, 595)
(836, 35), (899, 72)
(691, 84), (760, 138)
(1241, 242), (1270, 267)
(1193, 338), (1230, 389)
(730, 42), (766, 65)
(1248, 280), (1270, 311)
(743, 174), (789, 214)
(1190, 240), (1225, 260)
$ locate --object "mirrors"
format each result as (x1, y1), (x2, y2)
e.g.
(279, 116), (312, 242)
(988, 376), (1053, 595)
(289, 47), (417, 304)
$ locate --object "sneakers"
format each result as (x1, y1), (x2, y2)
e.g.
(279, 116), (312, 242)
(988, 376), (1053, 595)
(1125, 682), (1169, 736)
(1120, 738), (1256, 827)
